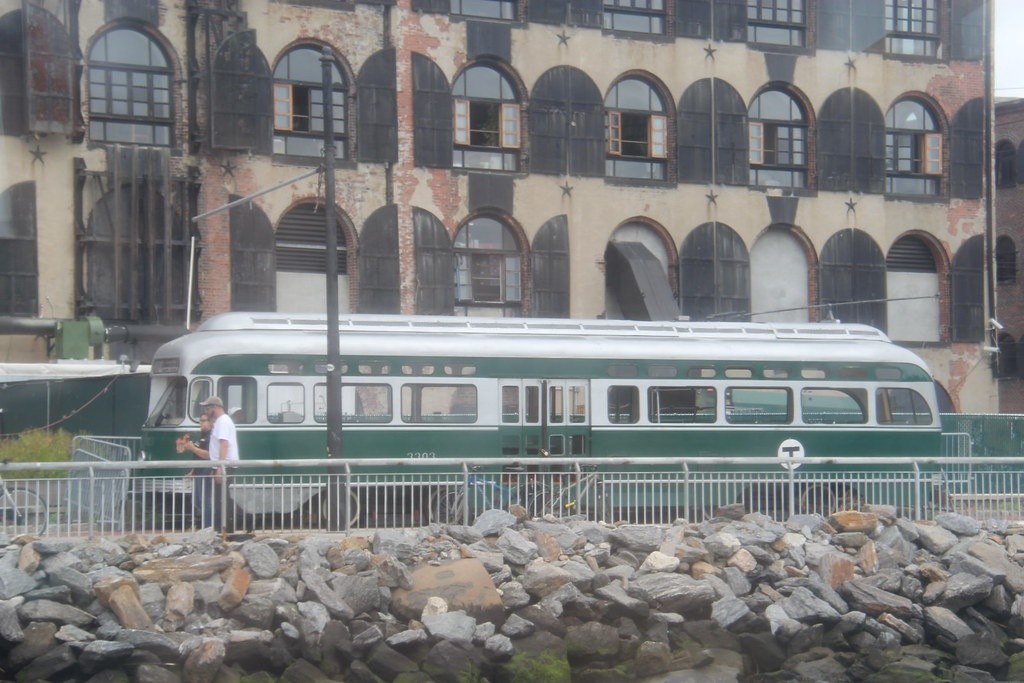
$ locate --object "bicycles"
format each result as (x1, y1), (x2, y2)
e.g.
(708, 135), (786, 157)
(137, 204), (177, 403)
(426, 465), (545, 522)
(496, 464), (611, 523)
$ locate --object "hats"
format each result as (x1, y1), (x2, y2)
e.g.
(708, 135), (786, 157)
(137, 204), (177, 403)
(199, 396), (224, 407)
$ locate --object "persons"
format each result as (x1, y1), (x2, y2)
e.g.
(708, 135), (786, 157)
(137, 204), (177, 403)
(199, 396), (240, 533)
(185, 413), (213, 530)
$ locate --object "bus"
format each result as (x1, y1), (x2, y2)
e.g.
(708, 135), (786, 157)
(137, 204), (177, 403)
(137, 308), (954, 519)
(0, 360), (358, 447)
